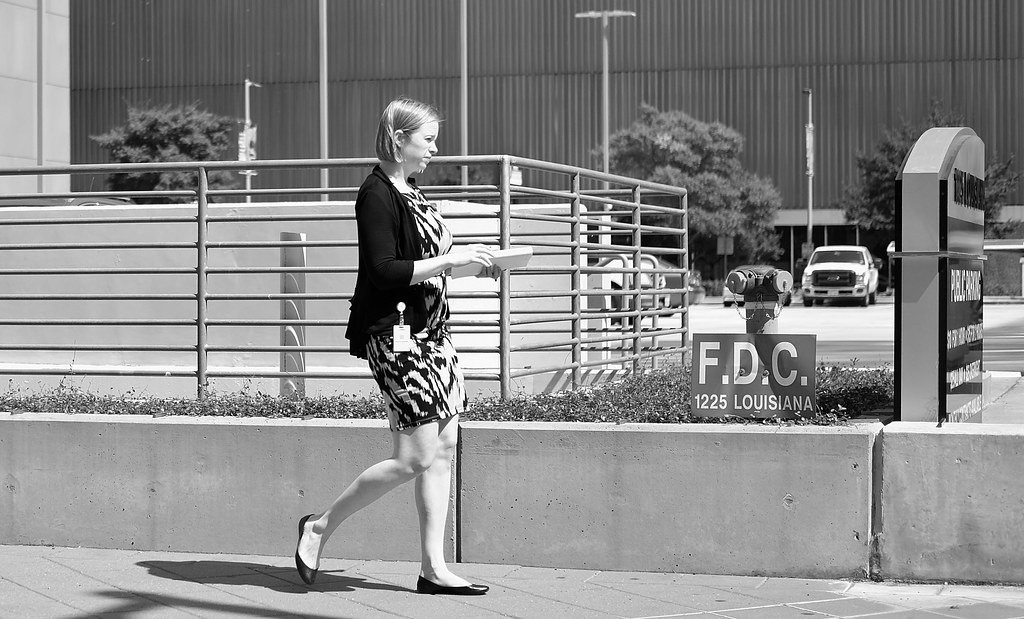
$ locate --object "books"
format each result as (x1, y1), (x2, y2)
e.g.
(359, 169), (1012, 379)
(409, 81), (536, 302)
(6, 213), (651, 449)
(449, 246), (533, 279)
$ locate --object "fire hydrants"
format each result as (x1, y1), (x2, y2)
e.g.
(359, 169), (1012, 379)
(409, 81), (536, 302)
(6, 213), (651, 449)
(725, 265), (795, 337)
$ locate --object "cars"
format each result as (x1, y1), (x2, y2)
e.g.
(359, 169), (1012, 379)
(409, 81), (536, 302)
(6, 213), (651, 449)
(800, 245), (880, 308)
(722, 265), (774, 308)
(590, 253), (699, 317)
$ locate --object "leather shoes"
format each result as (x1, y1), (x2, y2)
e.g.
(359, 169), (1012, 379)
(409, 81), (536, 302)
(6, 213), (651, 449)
(416, 574), (491, 598)
(294, 513), (320, 586)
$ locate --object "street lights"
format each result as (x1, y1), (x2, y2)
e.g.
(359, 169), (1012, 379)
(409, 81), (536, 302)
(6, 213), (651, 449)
(242, 78), (264, 202)
(573, 9), (636, 261)
(802, 87), (818, 265)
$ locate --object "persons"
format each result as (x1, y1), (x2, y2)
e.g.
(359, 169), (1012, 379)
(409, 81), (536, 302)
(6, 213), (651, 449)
(295, 95), (501, 595)
